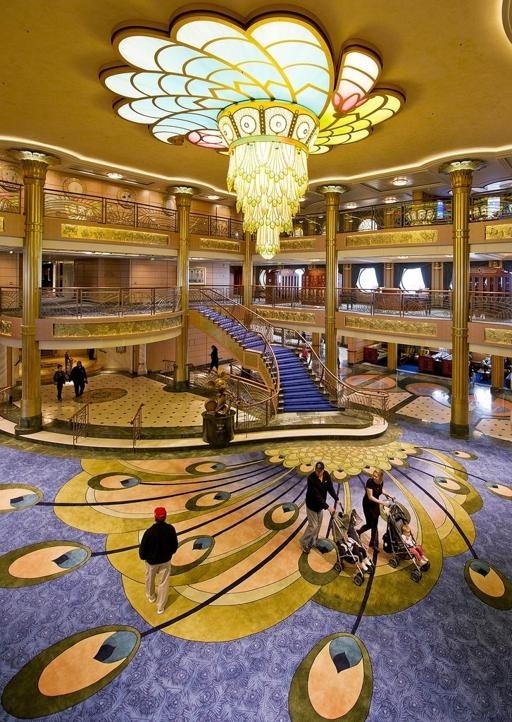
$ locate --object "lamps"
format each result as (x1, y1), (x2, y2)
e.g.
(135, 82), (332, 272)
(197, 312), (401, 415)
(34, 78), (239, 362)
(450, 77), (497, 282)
(98, 9), (405, 258)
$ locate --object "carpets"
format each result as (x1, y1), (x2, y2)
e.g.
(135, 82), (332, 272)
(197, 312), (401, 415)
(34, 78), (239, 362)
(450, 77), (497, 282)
(194, 306), (339, 412)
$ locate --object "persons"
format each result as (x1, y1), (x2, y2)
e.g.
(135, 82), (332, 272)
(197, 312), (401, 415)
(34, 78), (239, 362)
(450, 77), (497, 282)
(338, 529), (373, 572)
(354, 468), (396, 554)
(320, 338), (326, 369)
(401, 524), (430, 566)
(207, 343), (218, 375)
(300, 461), (342, 554)
(337, 341), (342, 369)
(52, 363), (68, 401)
(301, 331), (306, 343)
(301, 343), (309, 363)
(138, 507), (178, 616)
(65, 360), (89, 398)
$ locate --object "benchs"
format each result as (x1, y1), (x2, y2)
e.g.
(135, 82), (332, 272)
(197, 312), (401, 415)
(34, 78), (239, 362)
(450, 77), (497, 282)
(364, 342), (452, 376)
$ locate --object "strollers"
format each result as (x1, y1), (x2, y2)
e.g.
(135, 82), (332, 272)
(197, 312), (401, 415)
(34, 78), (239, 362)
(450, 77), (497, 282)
(326, 502), (374, 585)
(377, 495), (430, 583)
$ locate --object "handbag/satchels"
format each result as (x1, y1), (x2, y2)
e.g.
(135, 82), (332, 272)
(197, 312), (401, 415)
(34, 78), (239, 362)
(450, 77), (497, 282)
(383, 533), (392, 553)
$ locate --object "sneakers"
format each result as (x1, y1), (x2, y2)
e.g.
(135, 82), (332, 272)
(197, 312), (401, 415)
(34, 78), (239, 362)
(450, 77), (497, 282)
(145, 593), (164, 614)
(363, 561), (372, 571)
(369, 542), (381, 553)
(299, 538), (317, 554)
(418, 556), (429, 566)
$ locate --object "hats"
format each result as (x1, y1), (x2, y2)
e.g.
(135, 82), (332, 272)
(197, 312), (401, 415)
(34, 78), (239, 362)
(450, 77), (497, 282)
(155, 507), (166, 519)
(315, 462), (324, 471)
